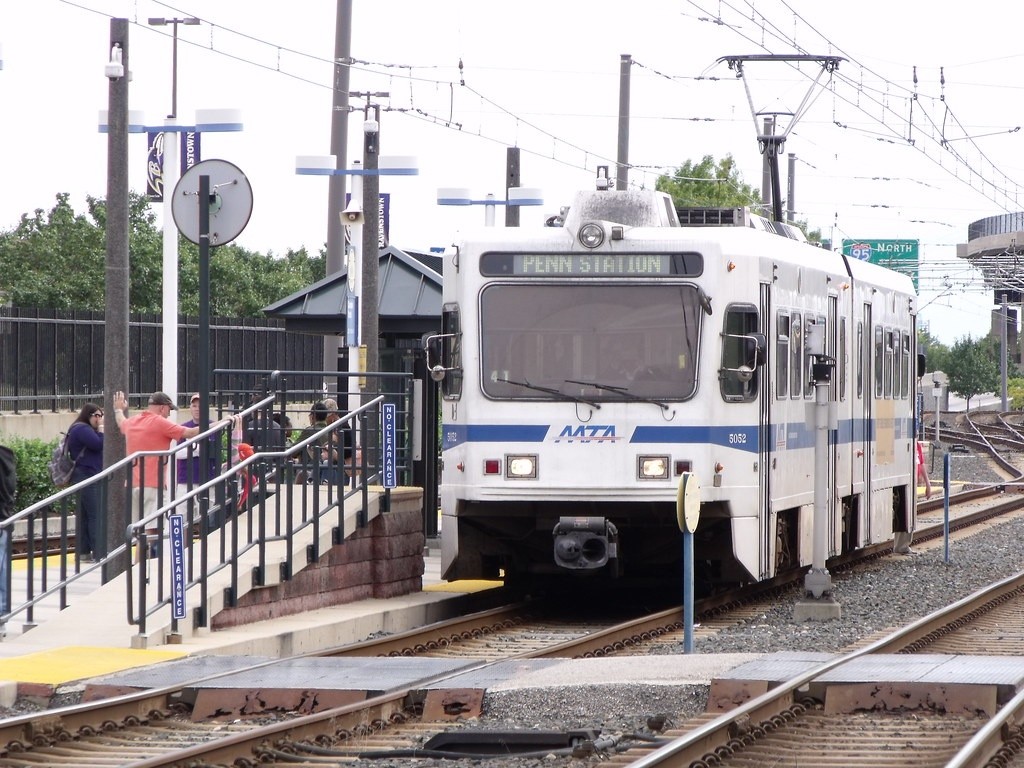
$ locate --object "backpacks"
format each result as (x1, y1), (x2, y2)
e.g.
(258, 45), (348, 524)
(52, 422), (96, 484)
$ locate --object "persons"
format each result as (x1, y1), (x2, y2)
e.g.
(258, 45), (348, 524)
(308, 440), (344, 484)
(0, 445), (17, 636)
(291, 401), (330, 466)
(65, 402), (106, 563)
(176, 393), (222, 549)
(332, 421), (356, 485)
(244, 397), (284, 455)
(323, 398), (343, 429)
(113, 391), (234, 565)
(270, 413), (294, 451)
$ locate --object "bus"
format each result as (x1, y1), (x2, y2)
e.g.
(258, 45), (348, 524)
(412, 187), (929, 590)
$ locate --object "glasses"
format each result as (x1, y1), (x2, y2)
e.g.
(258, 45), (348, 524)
(90, 414), (103, 418)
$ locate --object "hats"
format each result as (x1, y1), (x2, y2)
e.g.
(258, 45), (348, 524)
(323, 400), (338, 417)
(148, 392), (178, 411)
(190, 393), (200, 403)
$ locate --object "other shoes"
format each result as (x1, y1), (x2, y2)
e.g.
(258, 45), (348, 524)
(77, 552), (96, 562)
